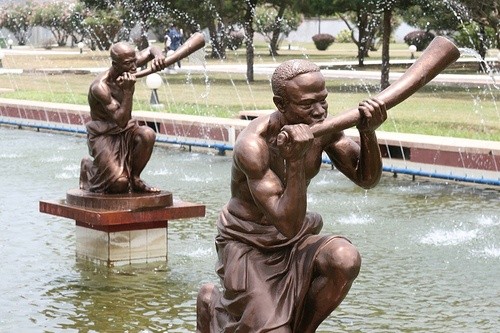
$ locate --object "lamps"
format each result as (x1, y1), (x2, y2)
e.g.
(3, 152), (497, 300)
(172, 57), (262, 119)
(409, 44), (416, 52)
(166, 50), (172, 57)
(146, 73), (163, 89)
(7, 39), (13, 45)
(78, 42), (85, 48)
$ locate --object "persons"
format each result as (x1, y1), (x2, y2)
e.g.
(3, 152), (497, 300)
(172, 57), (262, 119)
(78, 44), (166, 194)
(166, 22), (183, 72)
(195, 59), (394, 330)
